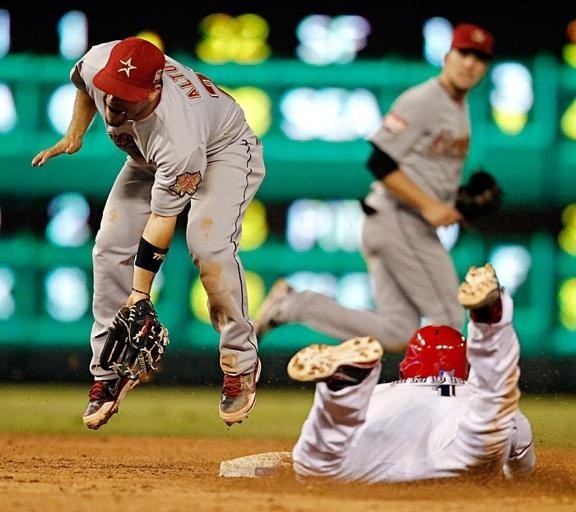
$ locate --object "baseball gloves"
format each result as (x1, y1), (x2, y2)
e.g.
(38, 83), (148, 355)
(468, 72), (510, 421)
(99, 300), (170, 383)
(455, 170), (503, 222)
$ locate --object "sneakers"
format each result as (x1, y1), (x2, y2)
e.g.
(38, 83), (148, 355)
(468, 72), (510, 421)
(82, 370), (141, 432)
(284, 332), (385, 383)
(252, 274), (296, 344)
(456, 261), (500, 324)
(218, 355), (263, 426)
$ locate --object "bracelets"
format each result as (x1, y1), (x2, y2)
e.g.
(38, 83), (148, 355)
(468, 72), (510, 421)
(130, 286), (151, 299)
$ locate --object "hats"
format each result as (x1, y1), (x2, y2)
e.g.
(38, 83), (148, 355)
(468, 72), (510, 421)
(92, 35), (166, 103)
(450, 22), (494, 56)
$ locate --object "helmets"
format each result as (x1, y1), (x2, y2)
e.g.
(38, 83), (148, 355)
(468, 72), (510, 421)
(399, 324), (471, 383)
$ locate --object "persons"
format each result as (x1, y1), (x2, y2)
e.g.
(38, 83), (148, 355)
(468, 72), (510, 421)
(254, 23), (505, 353)
(30, 37), (268, 428)
(286, 262), (537, 483)
